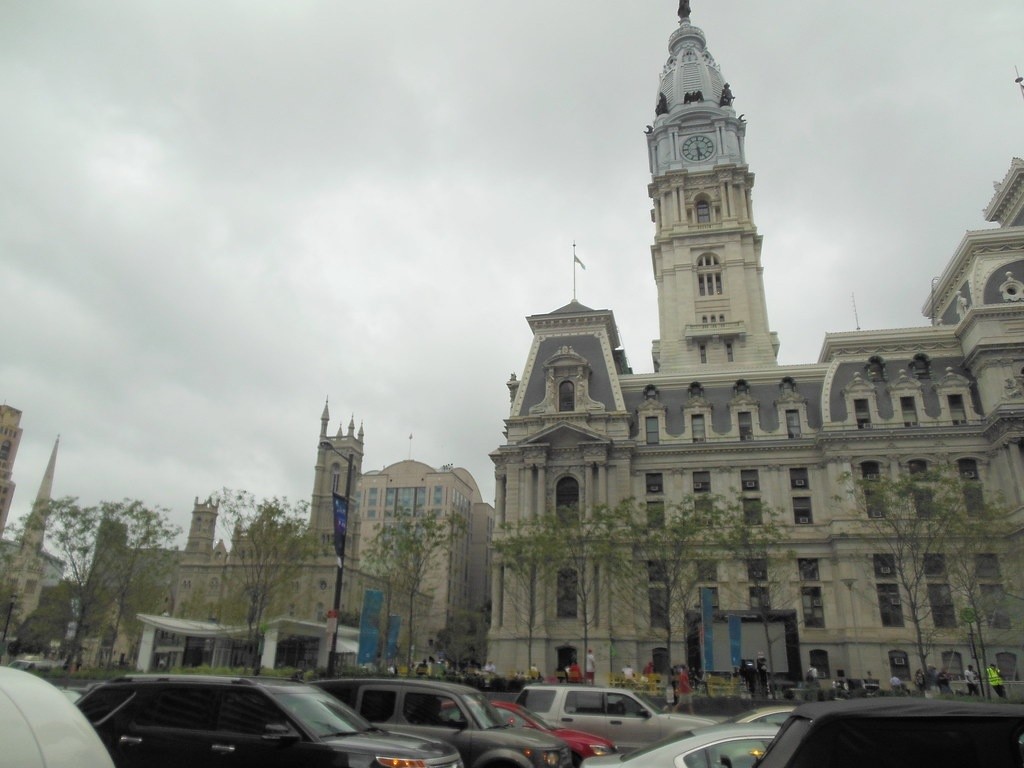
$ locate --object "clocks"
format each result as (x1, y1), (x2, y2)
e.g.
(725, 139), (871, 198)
(682, 135), (715, 162)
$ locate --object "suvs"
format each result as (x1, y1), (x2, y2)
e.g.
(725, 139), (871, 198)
(305, 676), (573, 768)
(754, 698), (1024, 767)
(65, 675), (464, 768)
(509, 684), (720, 754)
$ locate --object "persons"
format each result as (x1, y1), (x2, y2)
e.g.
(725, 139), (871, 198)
(889, 672), (911, 697)
(622, 661), (693, 714)
(964, 664), (982, 697)
(387, 649), (597, 684)
(915, 665), (957, 697)
(805, 660), (821, 687)
(985, 663), (1009, 700)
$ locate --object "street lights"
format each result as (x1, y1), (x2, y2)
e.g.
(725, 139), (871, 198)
(320, 440), (356, 682)
(2, 592), (17, 644)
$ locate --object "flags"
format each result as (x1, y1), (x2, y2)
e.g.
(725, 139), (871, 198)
(331, 493), (349, 556)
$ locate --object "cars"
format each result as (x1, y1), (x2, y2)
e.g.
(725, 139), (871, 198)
(6, 659), (60, 675)
(717, 705), (798, 759)
(440, 700), (619, 768)
(577, 722), (782, 768)
